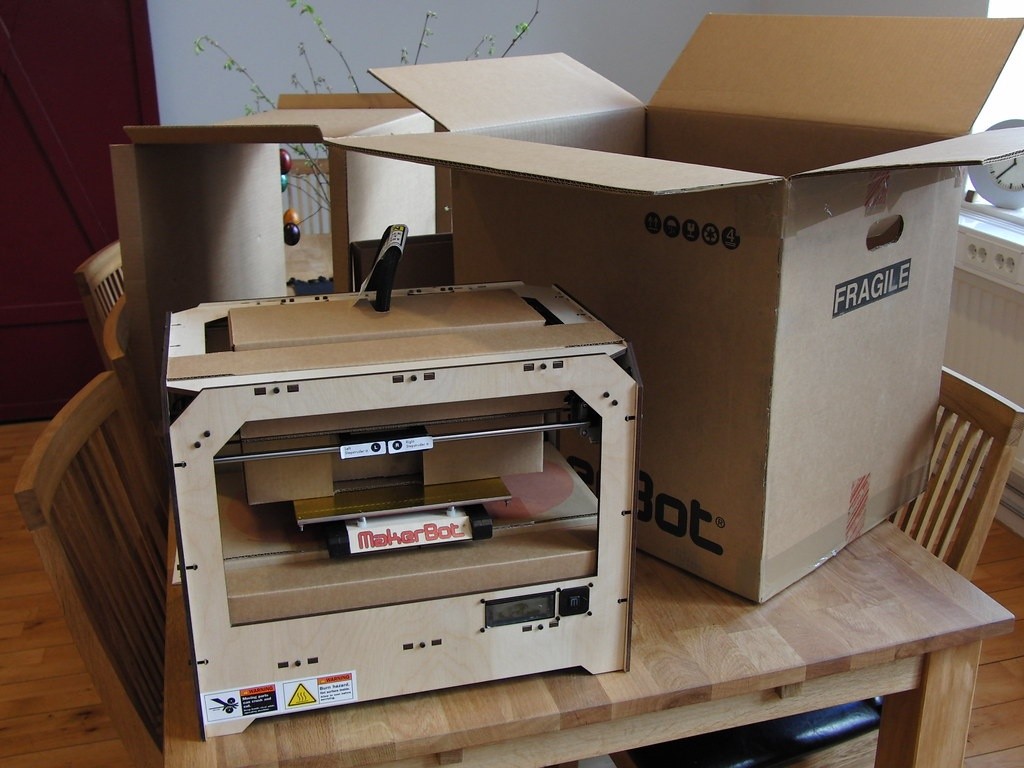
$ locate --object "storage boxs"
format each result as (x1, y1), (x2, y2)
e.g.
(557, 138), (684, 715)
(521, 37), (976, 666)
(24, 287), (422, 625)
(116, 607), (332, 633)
(320, 12), (1024, 608)
(110, 93), (451, 422)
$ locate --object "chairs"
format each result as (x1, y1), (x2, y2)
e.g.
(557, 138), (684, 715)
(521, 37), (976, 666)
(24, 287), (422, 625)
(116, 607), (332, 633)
(71, 239), (124, 368)
(13, 370), (165, 768)
(544, 365), (1024, 768)
(101, 292), (166, 496)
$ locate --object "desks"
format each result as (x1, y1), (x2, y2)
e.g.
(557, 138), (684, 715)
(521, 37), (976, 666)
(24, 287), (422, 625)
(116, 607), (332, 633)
(156, 482), (1014, 768)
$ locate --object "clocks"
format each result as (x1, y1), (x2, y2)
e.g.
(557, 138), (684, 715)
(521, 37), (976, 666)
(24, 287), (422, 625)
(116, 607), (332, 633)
(969, 119), (1024, 210)
